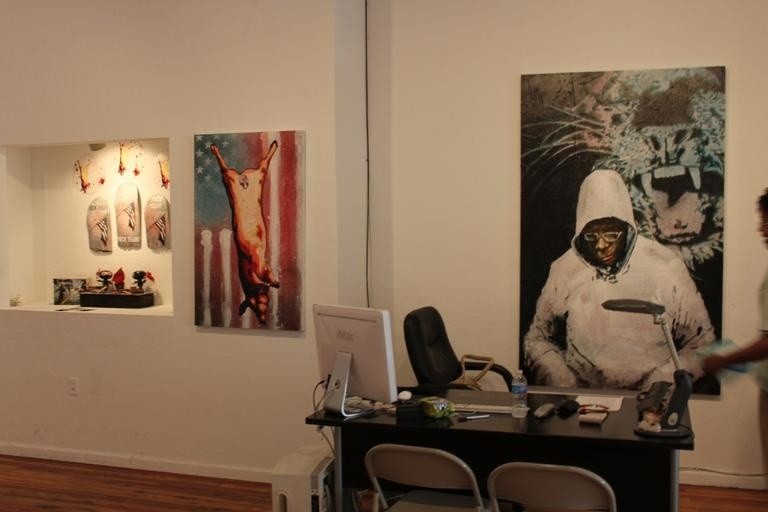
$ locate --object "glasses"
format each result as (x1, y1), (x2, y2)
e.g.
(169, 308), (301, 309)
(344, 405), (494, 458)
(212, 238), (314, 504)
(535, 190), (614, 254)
(576, 403), (609, 414)
(581, 227), (625, 242)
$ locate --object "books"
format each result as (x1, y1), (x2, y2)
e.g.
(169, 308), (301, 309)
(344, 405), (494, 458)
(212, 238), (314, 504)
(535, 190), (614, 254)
(445, 386), (514, 414)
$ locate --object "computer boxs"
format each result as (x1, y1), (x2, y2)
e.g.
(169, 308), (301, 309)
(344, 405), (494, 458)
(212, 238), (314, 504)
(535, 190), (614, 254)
(271, 444), (336, 512)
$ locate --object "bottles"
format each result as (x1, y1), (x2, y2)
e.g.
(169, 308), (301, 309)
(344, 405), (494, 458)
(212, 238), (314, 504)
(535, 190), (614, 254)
(510, 369), (528, 417)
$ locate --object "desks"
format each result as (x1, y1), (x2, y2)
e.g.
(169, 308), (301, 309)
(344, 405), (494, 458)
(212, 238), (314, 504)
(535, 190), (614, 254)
(305, 385), (695, 512)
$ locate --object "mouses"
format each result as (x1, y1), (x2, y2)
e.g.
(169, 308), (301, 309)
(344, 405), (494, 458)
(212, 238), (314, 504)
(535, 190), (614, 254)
(397, 391), (413, 402)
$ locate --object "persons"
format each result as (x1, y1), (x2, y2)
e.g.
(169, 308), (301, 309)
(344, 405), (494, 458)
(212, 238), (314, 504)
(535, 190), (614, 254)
(95, 269), (112, 292)
(522, 167), (722, 391)
(699, 184), (768, 486)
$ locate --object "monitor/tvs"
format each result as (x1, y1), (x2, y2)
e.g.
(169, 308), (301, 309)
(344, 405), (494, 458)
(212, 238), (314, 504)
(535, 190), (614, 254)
(312, 304), (398, 418)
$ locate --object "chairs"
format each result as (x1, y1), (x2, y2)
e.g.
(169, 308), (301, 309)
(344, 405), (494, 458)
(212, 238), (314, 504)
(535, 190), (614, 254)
(363, 443), (492, 512)
(403, 307), (514, 392)
(486, 462), (617, 511)
